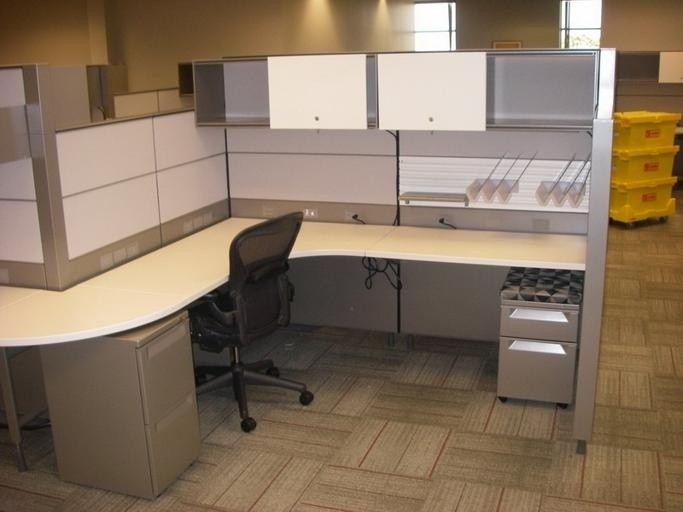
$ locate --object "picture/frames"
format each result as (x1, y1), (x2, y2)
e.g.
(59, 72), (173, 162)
(491, 40), (523, 48)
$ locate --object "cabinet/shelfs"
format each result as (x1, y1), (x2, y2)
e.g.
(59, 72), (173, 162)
(497, 267), (585, 409)
(40, 310), (207, 500)
(193, 49), (616, 131)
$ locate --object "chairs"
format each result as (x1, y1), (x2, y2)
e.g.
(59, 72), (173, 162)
(187, 212), (314, 432)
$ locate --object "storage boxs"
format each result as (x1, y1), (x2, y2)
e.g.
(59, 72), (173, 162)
(609, 110), (683, 228)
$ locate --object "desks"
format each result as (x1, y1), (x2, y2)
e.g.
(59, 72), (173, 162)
(0, 217), (587, 348)
